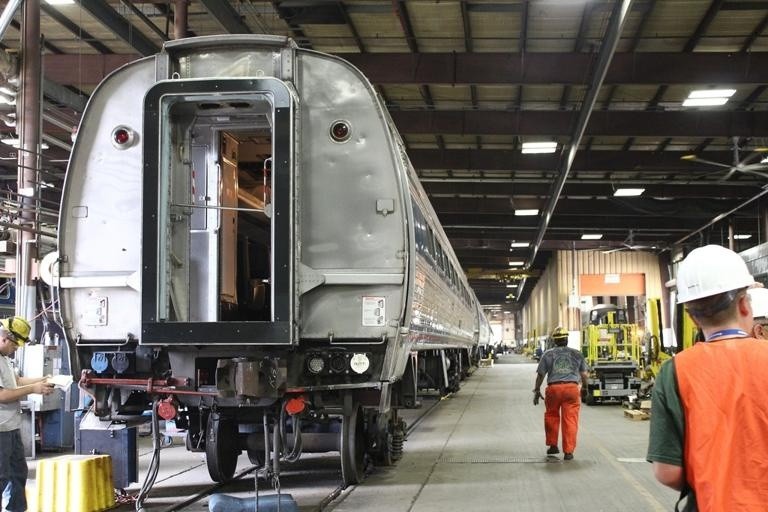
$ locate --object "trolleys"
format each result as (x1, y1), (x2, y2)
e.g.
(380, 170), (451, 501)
(157, 416), (187, 448)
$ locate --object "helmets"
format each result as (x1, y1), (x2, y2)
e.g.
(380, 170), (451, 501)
(675, 244), (764, 305)
(551, 326), (569, 340)
(1, 316), (31, 346)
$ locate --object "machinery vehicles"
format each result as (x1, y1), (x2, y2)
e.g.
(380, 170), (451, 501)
(578, 298), (646, 410)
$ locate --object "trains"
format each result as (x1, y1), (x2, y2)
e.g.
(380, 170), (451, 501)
(29, 30), (503, 486)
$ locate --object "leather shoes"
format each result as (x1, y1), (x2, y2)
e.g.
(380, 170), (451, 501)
(564, 453), (573, 460)
(547, 447), (559, 454)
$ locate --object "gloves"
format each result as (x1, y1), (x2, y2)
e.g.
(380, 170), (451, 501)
(532, 388), (544, 405)
(581, 388), (589, 404)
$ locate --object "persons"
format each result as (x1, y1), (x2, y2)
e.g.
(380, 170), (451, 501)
(742, 283), (768, 343)
(533, 325), (590, 459)
(0, 317), (54, 511)
(646, 243), (768, 510)
